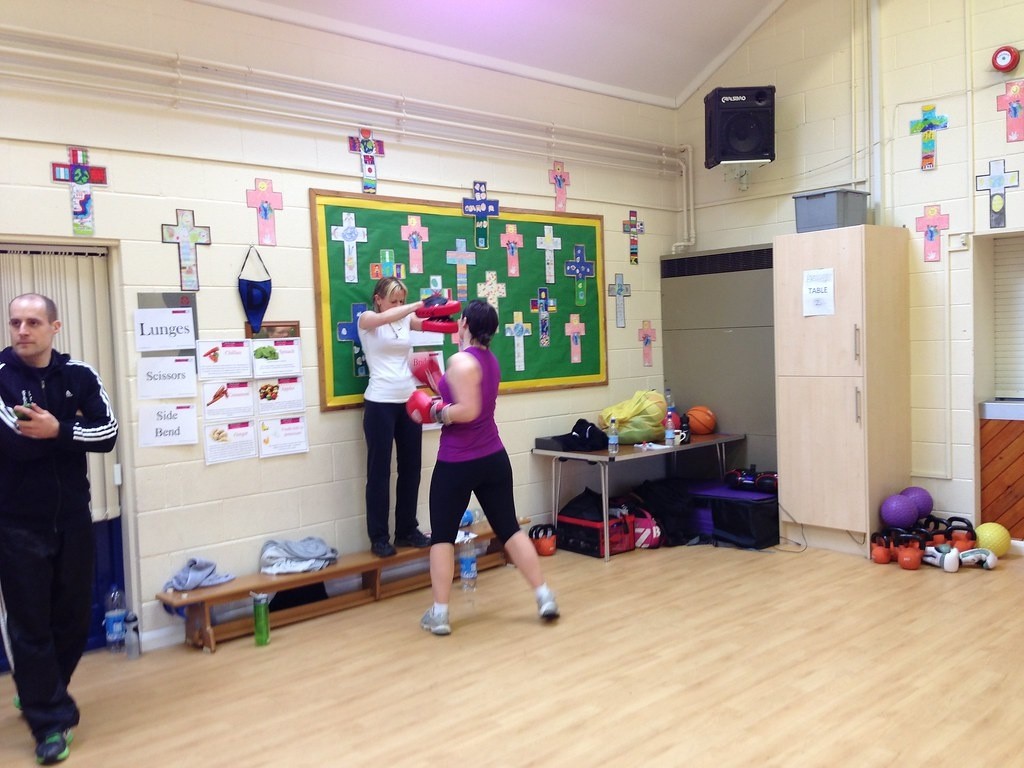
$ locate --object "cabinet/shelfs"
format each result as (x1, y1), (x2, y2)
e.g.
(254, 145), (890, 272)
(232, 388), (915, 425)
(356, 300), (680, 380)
(773, 223), (909, 560)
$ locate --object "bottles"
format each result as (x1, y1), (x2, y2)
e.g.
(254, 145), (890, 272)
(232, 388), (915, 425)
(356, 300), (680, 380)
(665, 389), (675, 407)
(459, 510), (483, 528)
(125, 613), (140, 659)
(642, 441), (646, 451)
(105, 585), (127, 652)
(253, 594), (269, 646)
(680, 414), (690, 444)
(665, 414), (674, 446)
(459, 532), (478, 592)
(609, 419), (619, 454)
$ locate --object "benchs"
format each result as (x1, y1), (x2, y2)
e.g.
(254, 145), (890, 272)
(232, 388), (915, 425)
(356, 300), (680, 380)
(154, 517), (531, 654)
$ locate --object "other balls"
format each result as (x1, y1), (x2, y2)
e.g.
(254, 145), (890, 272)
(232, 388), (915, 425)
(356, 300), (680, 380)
(899, 487), (933, 519)
(974, 522), (1011, 558)
(880, 495), (919, 528)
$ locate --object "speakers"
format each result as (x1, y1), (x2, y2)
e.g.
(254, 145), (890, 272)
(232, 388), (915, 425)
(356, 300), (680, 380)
(704, 85), (777, 169)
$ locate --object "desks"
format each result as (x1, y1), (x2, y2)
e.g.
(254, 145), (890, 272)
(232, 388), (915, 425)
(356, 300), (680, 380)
(530, 431), (747, 563)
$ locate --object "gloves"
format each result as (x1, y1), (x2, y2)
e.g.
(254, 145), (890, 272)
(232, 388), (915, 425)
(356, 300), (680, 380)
(406, 390), (450, 424)
(409, 352), (443, 393)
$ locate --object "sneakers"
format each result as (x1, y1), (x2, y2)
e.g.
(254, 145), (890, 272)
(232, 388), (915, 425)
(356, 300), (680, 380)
(13, 695), (22, 708)
(537, 593), (560, 621)
(394, 531), (431, 548)
(420, 608), (451, 635)
(36, 728), (73, 765)
(371, 542), (396, 558)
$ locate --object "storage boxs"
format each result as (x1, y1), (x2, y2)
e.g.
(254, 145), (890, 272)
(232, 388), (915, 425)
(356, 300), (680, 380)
(792, 188), (871, 233)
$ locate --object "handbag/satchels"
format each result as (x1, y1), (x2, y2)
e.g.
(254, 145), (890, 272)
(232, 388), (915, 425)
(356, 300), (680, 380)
(628, 506), (659, 549)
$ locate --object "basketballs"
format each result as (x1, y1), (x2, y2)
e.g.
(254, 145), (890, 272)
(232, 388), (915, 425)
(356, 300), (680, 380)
(685, 406), (716, 435)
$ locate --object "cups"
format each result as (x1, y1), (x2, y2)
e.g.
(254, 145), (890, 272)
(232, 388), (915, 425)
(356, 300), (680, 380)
(674, 430), (686, 446)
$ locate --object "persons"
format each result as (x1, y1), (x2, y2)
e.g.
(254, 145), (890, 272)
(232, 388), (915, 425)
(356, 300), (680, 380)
(0, 294), (121, 765)
(356, 275), (462, 555)
(403, 297), (560, 630)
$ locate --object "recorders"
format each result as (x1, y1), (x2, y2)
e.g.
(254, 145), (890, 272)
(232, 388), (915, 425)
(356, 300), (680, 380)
(724, 468), (778, 493)
